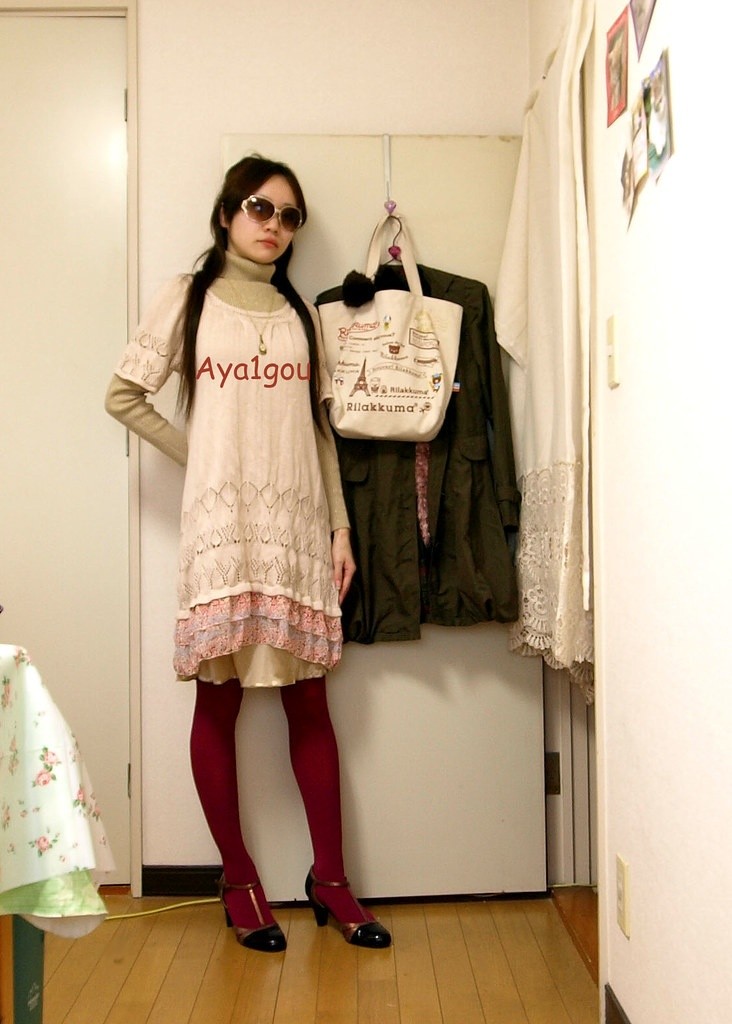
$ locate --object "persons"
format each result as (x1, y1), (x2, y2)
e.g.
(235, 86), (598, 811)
(106, 152), (393, 953)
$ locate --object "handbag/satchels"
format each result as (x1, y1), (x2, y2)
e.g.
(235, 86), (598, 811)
(316, 214), (464, 443)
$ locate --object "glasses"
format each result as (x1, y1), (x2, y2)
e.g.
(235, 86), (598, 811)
(240, 195), (302, 232)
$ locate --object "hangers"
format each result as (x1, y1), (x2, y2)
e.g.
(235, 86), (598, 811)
(381, 214), (401, 265)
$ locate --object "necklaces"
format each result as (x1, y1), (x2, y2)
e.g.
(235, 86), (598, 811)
(227, 275), (277, 353)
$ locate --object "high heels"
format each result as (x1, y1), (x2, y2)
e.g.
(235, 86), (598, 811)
(306, 864), (393, 950)
(218, 873), (287, 953)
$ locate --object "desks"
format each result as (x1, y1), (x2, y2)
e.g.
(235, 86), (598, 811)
(1, 643), (117, 1024)
